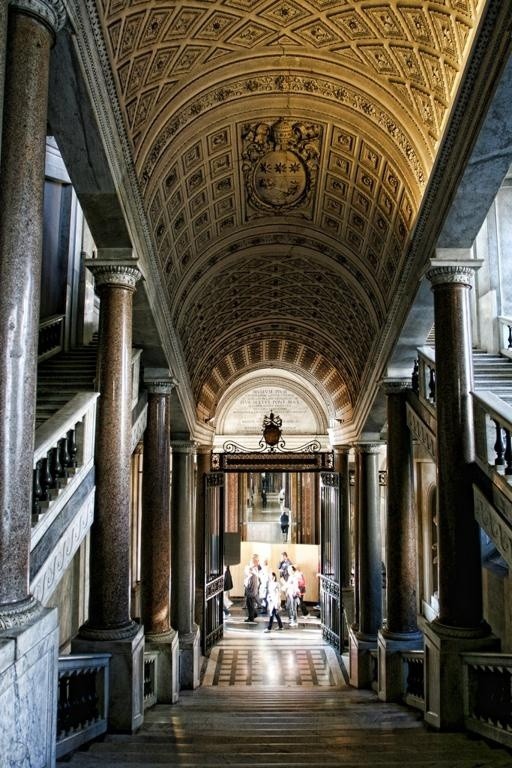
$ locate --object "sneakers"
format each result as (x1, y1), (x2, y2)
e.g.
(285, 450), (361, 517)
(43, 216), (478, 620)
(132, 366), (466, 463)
(224, 613), (232, 619)
(245, 612), (311, 633)
(313, 605), (320, 610)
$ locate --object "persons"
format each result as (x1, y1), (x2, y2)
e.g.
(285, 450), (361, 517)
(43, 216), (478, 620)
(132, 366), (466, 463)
(280, 512), (289, 542)
(262, 489), (267, 508)
(223, 551), (320, 633)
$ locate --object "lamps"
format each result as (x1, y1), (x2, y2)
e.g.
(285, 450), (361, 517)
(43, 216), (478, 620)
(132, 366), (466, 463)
(259, 408), (285, 453)
